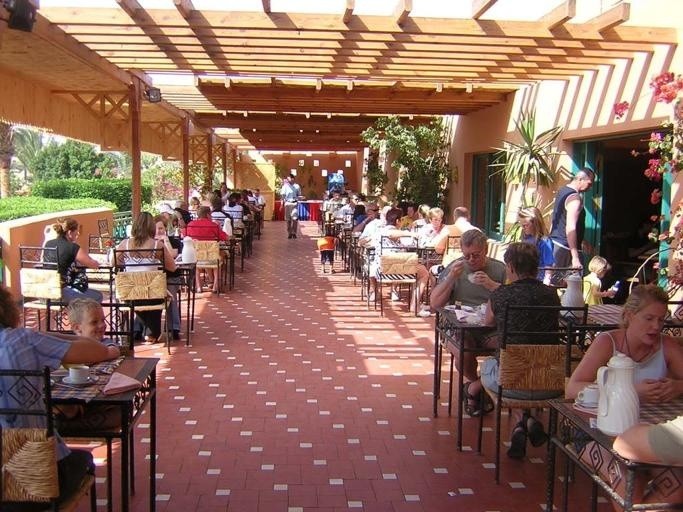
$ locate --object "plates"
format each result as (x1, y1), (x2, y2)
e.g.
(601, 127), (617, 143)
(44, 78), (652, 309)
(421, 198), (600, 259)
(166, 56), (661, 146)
(574, 397), (597, 408)
(445, 305), (472, 314)
(61, 375), (99, 386)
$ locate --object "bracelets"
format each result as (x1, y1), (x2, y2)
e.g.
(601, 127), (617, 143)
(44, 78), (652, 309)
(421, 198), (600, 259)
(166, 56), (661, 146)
(443, 276), (454, 285)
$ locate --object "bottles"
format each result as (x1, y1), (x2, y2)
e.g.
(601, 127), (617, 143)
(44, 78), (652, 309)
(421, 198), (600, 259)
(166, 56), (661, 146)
(606, 280), (619, 299)
(454, 301), (461, 310)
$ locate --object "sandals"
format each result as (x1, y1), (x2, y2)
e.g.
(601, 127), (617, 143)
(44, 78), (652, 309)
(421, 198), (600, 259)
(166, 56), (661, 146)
(463, 382), (495, 416)
(522, 415), (548, 448)
(507, 424), (526, 460)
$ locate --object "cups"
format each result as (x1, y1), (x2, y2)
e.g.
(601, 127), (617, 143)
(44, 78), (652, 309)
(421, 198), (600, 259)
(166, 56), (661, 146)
(165, 223), (185, 238)
(467, 274), (477, 284)
(68, 365), (90, 381)
(577, 385), (599, 404)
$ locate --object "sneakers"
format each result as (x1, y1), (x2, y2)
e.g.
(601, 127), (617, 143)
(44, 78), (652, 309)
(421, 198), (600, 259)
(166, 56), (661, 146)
(127, 330), (180, 345)
(369, 291), (430, 318)
(322, 269), (336, 274)
(288, 234), (297, 239)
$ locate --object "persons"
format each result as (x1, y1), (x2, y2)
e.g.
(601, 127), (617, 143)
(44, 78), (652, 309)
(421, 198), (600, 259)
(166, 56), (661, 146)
(0, 285), (118, 511)
(63, 297), (119, 361)
(429, 229), (508, 418)
(40, 217), (104, 306)
(481, 239), (558, 461)
(564, 283), (683, 404)
(612, 413), (683, 470)
(316, 188), (486, 319)
(332, 182), (341, 192)
(108, 177), (266, 343)
(281, 176), (287, 221)
(279, 173), (302, 239)
(582, 254), (619, 306)
(549, 167), (593, 342)
(517, 206), (554, 286)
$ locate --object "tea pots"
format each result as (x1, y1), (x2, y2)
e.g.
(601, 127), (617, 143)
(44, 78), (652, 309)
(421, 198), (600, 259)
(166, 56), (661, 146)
(561, 272), (592, 318)
(594, 352), (639, 436)
(180, 236), (198, 262)
(222, 217), (232, 236)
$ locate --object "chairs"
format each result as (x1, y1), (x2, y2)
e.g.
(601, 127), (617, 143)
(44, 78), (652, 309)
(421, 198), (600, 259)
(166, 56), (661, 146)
(352, 237), (378, 311)
(173, 196), (265, 297)
(87, 232), (118, 254)
(445, 235), (463, 267)
(18, 243), (65, 331)
(477, 301), (588, 487)
(0, 366), (96, 512)
(320, 210), (359, 270)
(536, 267), (584, 301)
(98, 219), (109, 237)
(562, 322), (623, 510)
(374, 234), (420, 320)
(659, 325), (682, 336)
(45, 298), (137, 511)
(112, 247), (173, 356)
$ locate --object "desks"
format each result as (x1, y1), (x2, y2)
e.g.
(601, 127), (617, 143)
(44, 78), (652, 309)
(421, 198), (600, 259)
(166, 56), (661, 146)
(274, 199), (326, 222)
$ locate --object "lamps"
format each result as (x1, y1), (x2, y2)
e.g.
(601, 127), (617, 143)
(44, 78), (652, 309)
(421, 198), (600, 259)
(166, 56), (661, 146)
(142, 85), (163, 103)
(0, 0), (38, 31)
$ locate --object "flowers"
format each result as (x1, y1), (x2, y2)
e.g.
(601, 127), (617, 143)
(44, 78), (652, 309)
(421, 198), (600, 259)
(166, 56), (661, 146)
(612, 68), (683, 287)
